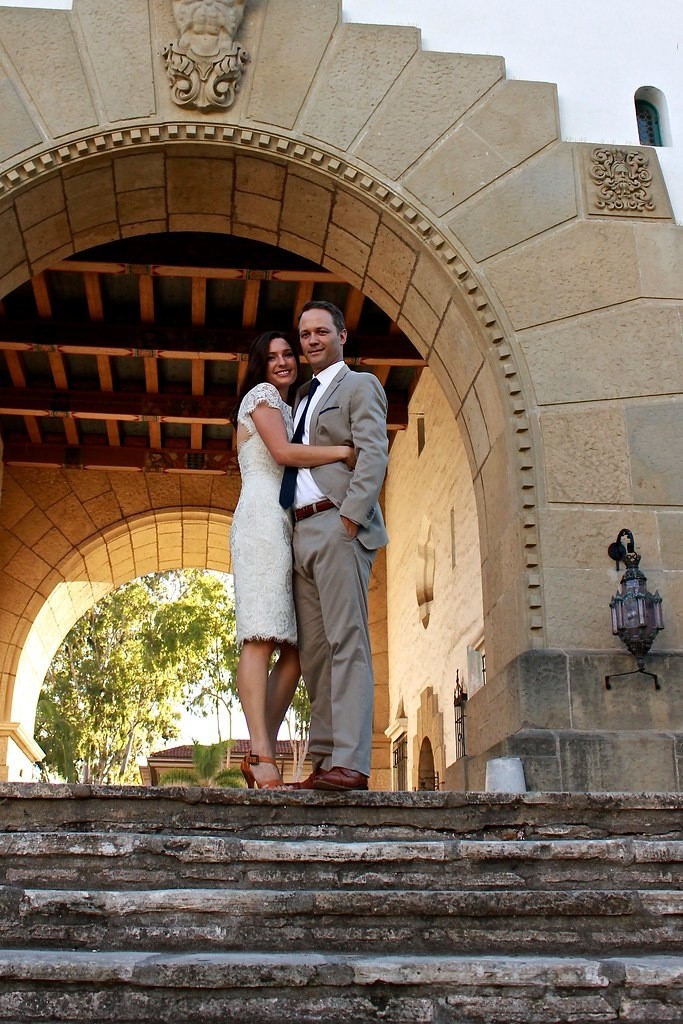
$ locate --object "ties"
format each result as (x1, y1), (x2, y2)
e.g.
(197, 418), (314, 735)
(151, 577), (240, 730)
(279, 378), (320, 509)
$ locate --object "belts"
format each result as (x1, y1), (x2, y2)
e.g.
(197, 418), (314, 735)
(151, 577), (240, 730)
(294, 499), (336, 522)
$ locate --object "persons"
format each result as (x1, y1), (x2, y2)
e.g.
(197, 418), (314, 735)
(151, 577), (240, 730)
(285, 299), (391, 791)
(228, 327), (357, 791)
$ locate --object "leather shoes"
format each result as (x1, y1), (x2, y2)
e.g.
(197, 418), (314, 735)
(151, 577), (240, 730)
(293, 768), (368, 791)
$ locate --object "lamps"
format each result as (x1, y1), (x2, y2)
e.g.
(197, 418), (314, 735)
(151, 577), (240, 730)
(606, 528), (665, 692)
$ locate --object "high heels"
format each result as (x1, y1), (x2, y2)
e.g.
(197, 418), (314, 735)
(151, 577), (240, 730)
(241, 751), (284, 789)
